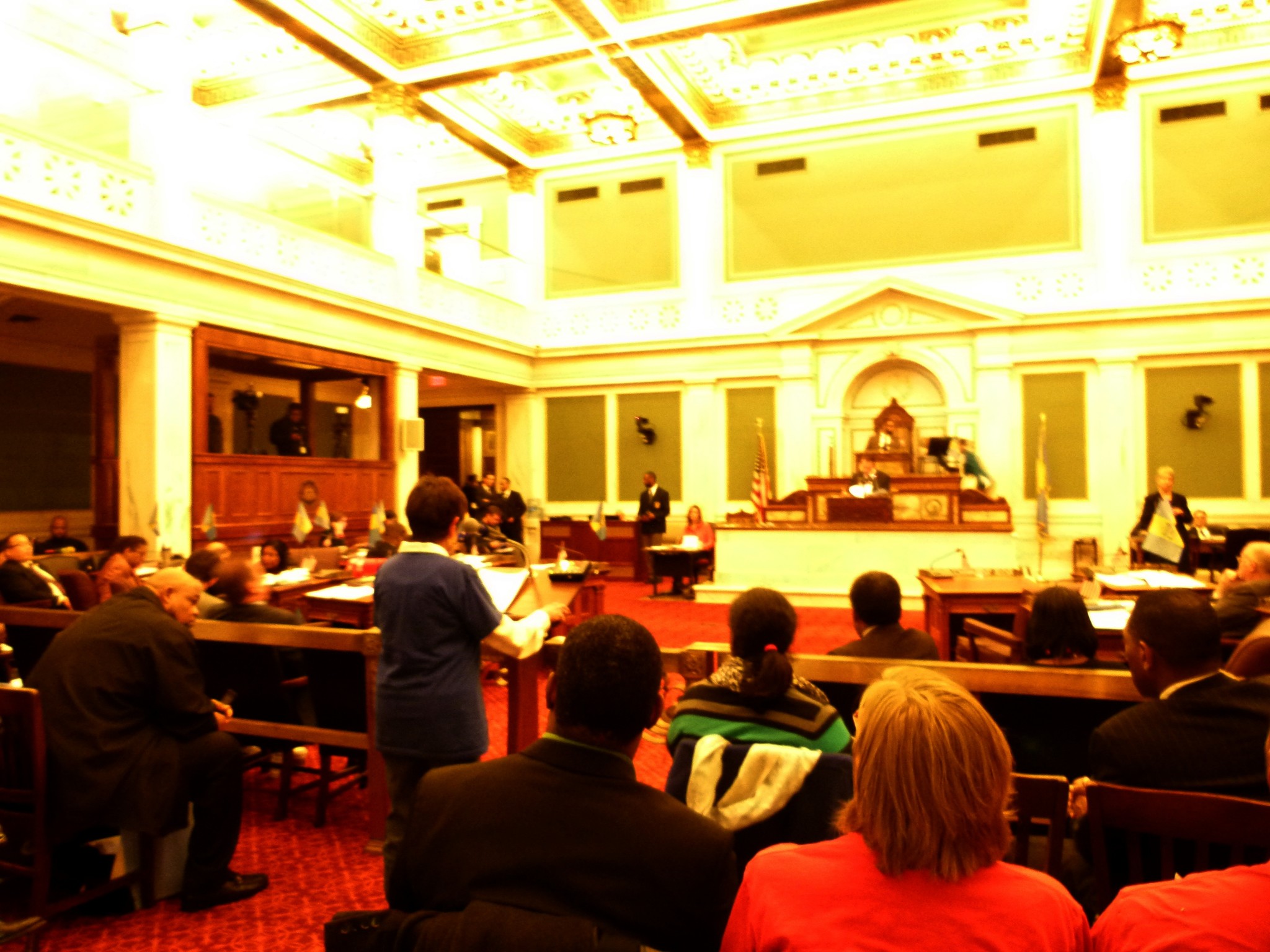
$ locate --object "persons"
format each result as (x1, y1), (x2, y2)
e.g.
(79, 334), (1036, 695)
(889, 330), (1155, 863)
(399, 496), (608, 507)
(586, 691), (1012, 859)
(1091, 723), (1270, 952)
(937, 435), (993, 495)
(254, 538), (290, 577)
(365, 461), (525, 563)
(0, 531), (74, 610)
(1126, 463), (1200, 577)
(662, 503), (716, 595)
(175, 547), (228, 615)
(1207, 541), (1270, 625)
(368, 470), (573, 834)
(207, 550), (311, 624)
(826, 570), (943, 664)
(204, 540), (232, 564)
(1071, 584), (1270, 879)
(664, 587), (855, 755)
(712, 663), (1093, 952)
(317, 505), (352, 547)
(267, 401), (311, 457)
(867, 417), (902, 452)
(206, 390), (225, 454)
(95, 534), (149, 604)
(849, 453), (891, 498)
(1018, 584), (1114, 673)
(34, 515), (92, 555)
(631, 467), (670, 585)
(1187, 507), (1214, 540)
(384, 609), (741, 952)
(28, 566), (272, 914)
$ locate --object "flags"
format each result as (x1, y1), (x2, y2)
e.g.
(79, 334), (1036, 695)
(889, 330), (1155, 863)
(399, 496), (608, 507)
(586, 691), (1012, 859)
(291, 501), (315, 543)
(1027, 423), (1051, 547)
(198, 504), (216, 541)
(314, 499), (333, 531)
(750, 431), (774, 525)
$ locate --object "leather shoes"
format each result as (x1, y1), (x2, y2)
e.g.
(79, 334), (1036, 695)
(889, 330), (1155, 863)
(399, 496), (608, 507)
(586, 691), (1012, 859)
(180, 872), (268, 912)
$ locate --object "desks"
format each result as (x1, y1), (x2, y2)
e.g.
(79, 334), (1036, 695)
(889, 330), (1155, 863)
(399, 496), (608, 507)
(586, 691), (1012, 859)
(502, 572), (606, 758)
(826, 497), (895, 524)
(855, 451), (913, 476)
(918, 564), (1218, 665)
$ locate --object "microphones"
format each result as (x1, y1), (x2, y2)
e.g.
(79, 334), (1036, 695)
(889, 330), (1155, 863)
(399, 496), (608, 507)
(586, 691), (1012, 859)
(461, 517), (507, 545)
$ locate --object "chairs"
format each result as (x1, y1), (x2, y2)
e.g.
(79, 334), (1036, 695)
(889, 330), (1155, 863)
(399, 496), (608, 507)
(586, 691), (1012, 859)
(0, 682), (161, 931)
(1003, 771), (1066, 883)
(0, 546), (387, 630)
(1067, 778), (1269, 906)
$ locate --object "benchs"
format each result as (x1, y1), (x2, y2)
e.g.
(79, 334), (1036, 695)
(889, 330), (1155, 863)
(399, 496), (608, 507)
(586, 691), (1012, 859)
(682, 643), (1153, 777)
(1, 604), (392, 852)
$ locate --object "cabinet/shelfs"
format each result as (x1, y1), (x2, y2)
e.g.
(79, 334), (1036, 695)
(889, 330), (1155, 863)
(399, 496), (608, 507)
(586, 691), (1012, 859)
(539, 518), (640, 566)
